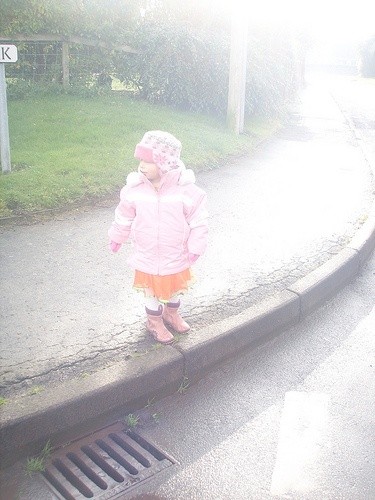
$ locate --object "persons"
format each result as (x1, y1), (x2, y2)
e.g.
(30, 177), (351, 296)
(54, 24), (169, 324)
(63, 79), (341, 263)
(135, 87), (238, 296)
(108, 130), (212, 347)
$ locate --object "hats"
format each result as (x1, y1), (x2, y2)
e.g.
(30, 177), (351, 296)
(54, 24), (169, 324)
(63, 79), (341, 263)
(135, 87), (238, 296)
(133, 130), (183, 172)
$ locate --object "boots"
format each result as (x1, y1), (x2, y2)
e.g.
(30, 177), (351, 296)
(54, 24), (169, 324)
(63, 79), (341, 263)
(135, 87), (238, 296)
(162, 299), (191, 335)
(145, 304), (175, 346)
